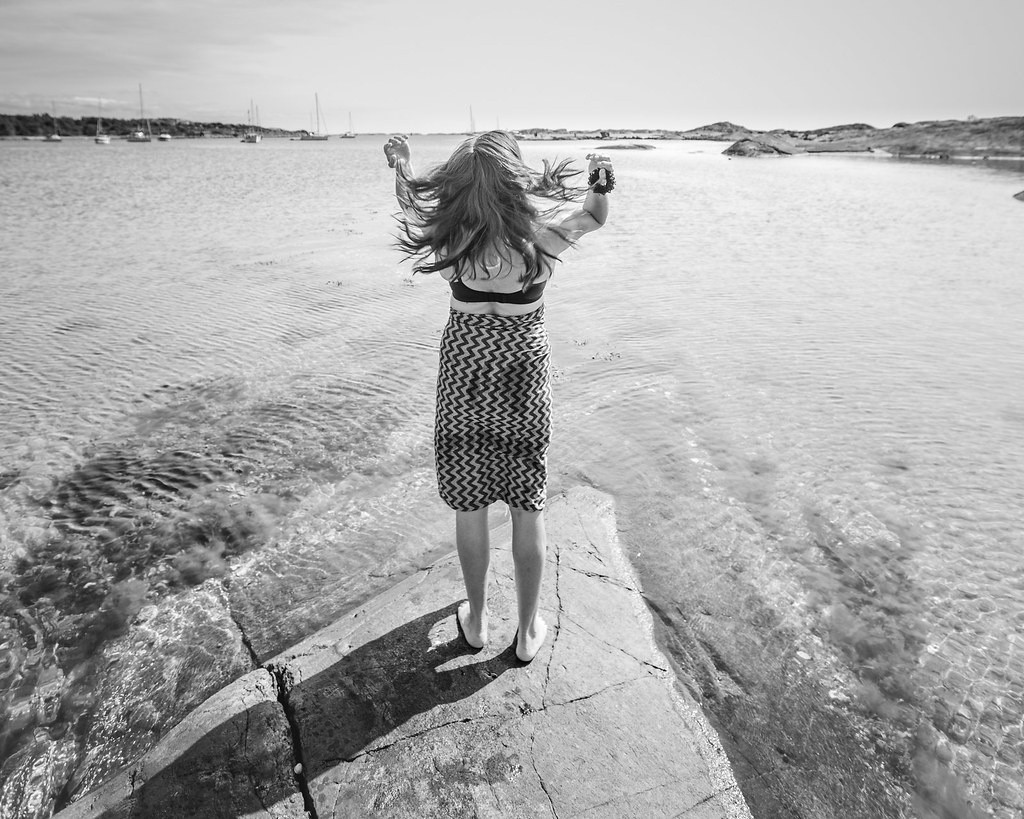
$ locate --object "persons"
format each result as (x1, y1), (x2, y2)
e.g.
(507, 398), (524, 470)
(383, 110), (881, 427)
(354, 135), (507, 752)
(382, 127), (615, 662)
